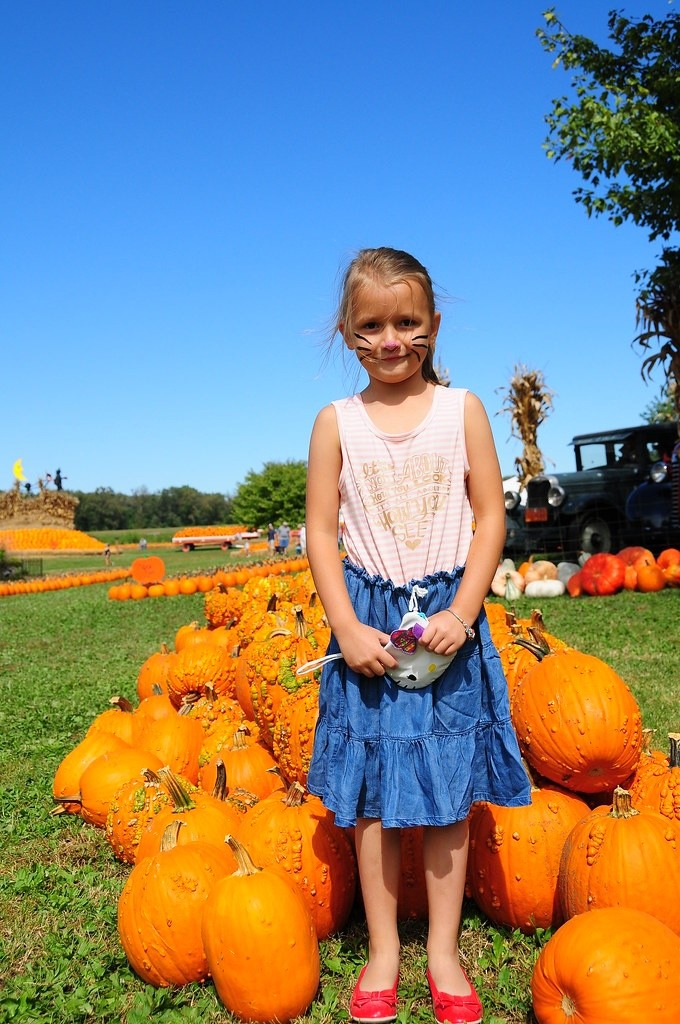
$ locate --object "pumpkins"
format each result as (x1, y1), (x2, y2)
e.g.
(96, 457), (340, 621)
(0, 526), (680, 1024)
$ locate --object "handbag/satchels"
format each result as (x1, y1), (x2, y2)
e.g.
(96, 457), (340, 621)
(296, 612), (457, 689)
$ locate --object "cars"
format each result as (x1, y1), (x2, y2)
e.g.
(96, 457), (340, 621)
(497, 421), (680, 564)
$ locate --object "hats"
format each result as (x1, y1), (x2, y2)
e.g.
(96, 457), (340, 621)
(283, 521), (288, 525)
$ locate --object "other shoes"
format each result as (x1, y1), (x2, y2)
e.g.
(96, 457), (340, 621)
(426, 965), (482, 1024)
(350, 964), (399, 1023)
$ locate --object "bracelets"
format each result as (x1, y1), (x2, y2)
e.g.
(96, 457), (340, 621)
(446, 608), (475, 640)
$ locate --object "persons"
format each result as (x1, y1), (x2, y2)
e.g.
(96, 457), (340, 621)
(55, 469), (67, 491)
(305, 246), (533, 1024)
(140, 537), (147, 550)
(104, 544), (114, 566)
(296, 523), (306, 555)
(267, 522), (291, 556)
